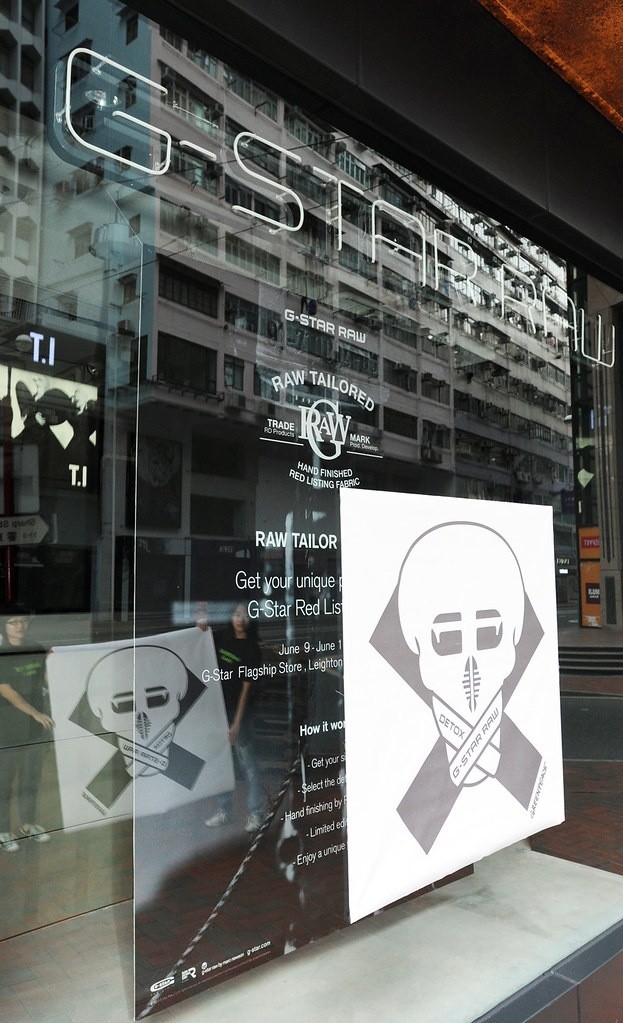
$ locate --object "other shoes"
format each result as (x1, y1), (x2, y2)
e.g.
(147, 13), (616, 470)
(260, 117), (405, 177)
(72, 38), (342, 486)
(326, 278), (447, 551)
(20, 822), (49, 842)
(0, 832), (20, 853)
(205, 808), (233, 827)
(246, 811), (262, 832)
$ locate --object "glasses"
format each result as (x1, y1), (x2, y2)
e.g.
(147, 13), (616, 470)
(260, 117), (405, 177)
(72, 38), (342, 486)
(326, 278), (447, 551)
(6, 616), (30, 625)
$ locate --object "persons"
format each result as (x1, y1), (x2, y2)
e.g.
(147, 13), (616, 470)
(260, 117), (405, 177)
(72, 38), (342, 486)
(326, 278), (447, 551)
(0, 606), (56, 853)
(197, 601), (265, 831)
